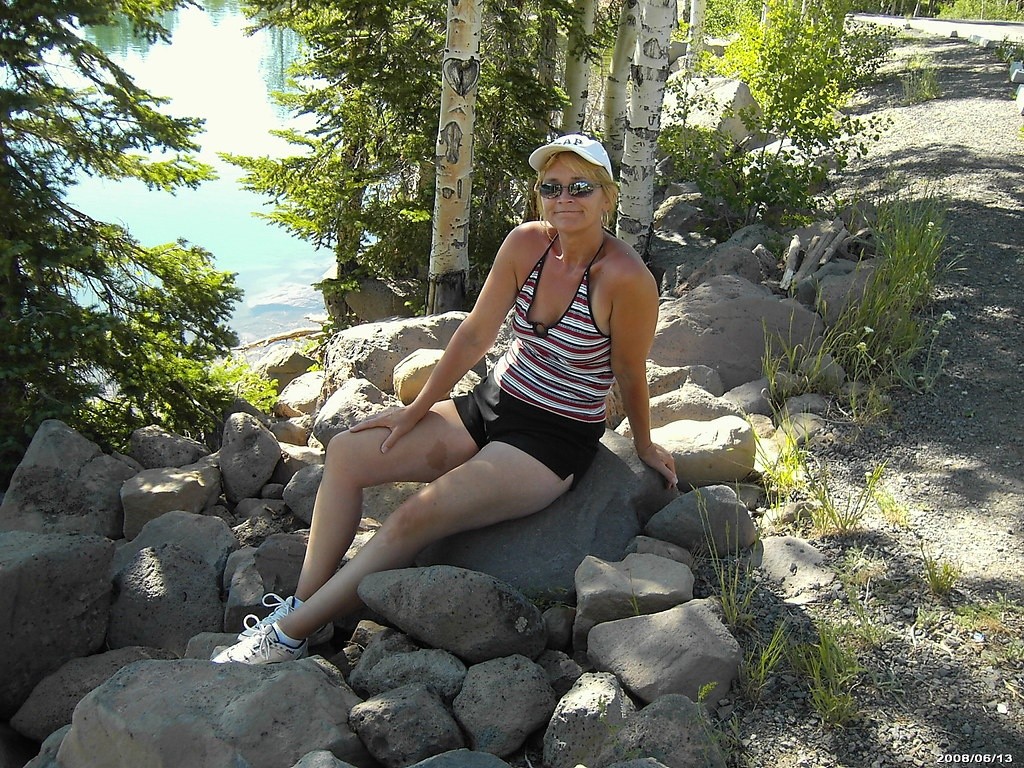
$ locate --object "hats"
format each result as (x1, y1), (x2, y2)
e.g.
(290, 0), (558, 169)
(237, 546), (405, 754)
(528, 133), (613, 181)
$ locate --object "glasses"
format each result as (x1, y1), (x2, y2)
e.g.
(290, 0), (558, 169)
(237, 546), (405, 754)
(537, 182), (602, 199)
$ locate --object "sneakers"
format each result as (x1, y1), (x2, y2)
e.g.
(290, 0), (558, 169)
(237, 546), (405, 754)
(237, 593), (334, 645)
(211, 614), (308, 665)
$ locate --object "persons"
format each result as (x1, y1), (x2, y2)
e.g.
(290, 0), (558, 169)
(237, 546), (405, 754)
(204, 133), (680, 667)
